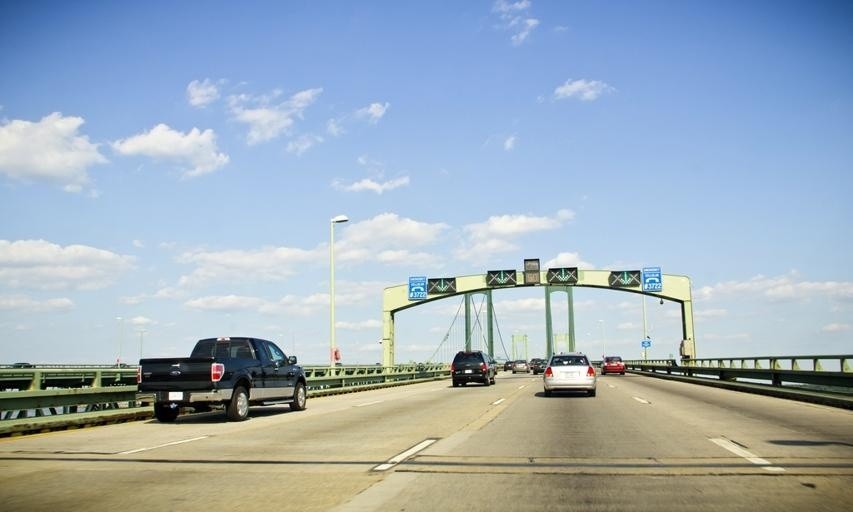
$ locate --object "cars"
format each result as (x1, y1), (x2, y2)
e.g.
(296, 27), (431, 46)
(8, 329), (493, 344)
(543, 354), (596, 396)
(504, 352), (549, 374)
(601, 356), (625, 375)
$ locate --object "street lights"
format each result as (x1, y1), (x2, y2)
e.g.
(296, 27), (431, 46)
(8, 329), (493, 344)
(597, 320), (605, 356)
(480, 309), (487, 351)
(330, 215), (350, 377)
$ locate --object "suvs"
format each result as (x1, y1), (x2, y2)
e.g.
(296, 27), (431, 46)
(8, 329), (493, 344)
(450, 351), (496, 386)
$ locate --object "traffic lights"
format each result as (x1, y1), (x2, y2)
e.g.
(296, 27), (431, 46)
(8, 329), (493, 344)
(428, 277), (456, 294)
(609, 271), (641, 286)
(522, 258), (541, 284)
(546, 268), (578, 283)
(487, 269), (517, 286)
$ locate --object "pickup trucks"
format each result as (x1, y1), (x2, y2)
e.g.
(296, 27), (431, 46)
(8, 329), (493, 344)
(135, 337), (308, 423)
